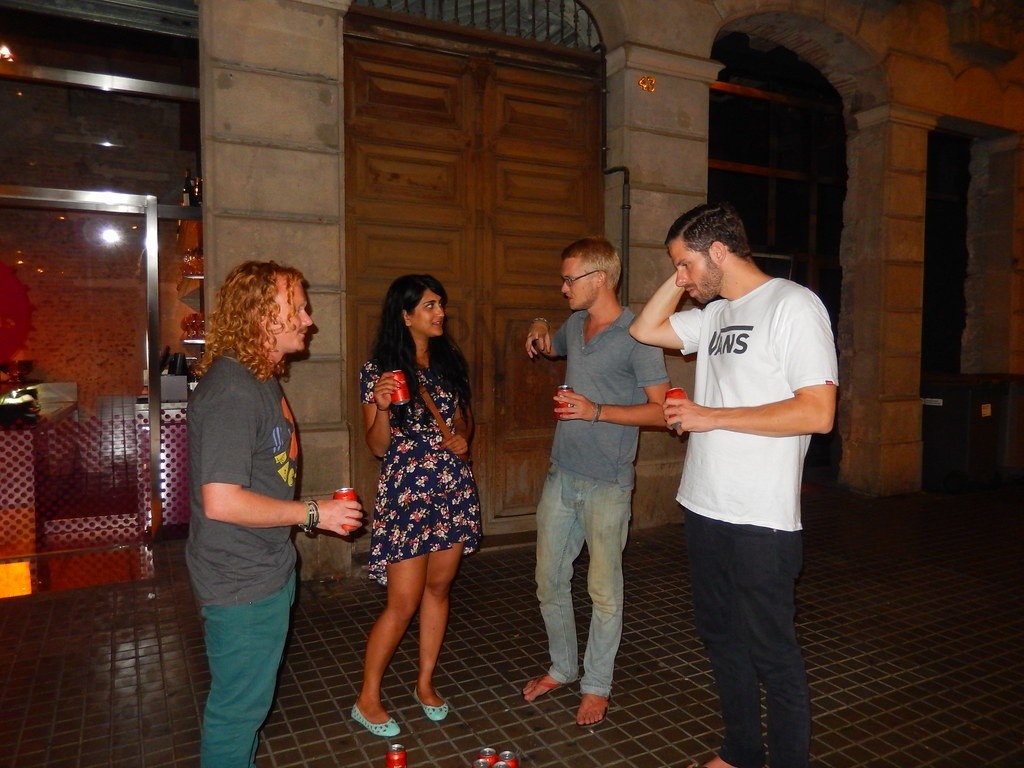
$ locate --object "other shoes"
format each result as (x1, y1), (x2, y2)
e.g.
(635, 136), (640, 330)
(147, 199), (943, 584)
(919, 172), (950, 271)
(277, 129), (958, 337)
(351, 701), (400, 737)
(695, 761), (710, 768)
(414, 687), (448, 720)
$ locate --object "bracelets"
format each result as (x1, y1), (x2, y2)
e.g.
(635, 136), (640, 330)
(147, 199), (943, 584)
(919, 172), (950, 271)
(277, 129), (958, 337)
(534, 316), (551, 329)
(377, 407), (389, 411)
(298, 498), (319, 534)
(592, 402), (601, 423)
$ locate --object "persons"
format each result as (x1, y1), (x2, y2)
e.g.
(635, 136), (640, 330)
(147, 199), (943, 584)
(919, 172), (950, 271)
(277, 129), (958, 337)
(349, 274), (483, 737)
(521, 236), (673, 726)
(628, 202), (840, 768)
(184, 261), (365, 768)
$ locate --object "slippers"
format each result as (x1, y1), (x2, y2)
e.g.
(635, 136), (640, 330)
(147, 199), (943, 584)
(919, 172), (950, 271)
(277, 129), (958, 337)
(577, 694), (611, 729)
(521, 672), (566, 702)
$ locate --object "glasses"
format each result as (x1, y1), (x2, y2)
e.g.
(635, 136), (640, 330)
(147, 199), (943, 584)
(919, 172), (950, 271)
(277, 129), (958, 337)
(560, 269), (599, 287)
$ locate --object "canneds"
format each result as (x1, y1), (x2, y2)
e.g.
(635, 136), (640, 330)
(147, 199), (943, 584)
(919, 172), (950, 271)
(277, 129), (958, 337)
(333, 487), (358, 532)
(554, 385), (574, 419)
(385, 744), (407, 768)
(389, 370), (410, 405)
(471, 748), (519, 768)
(665, 387), (689, 429)
